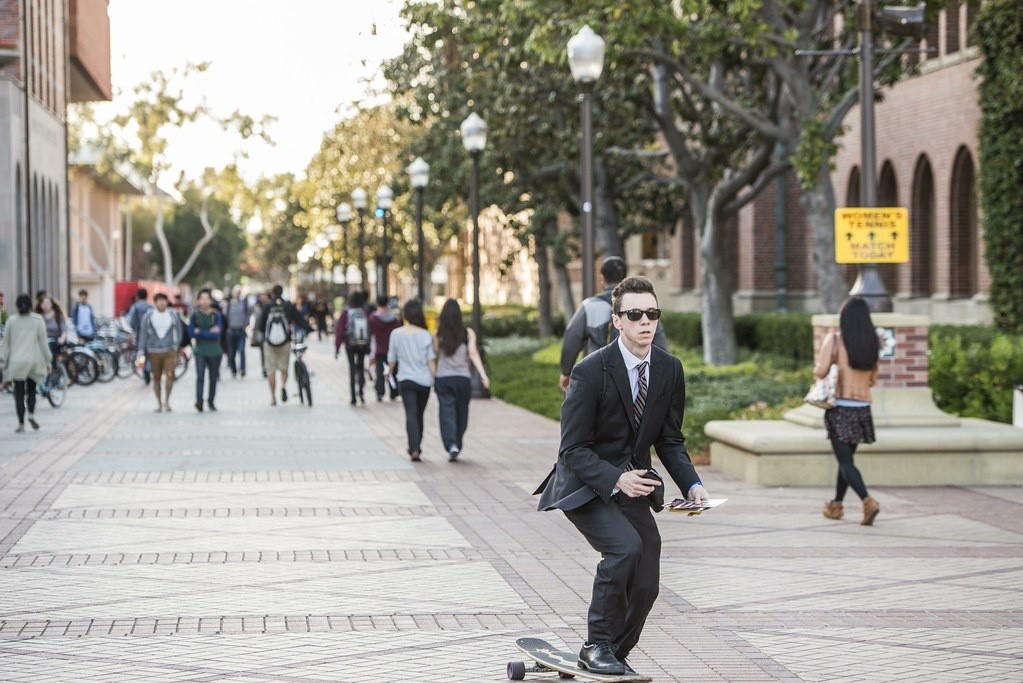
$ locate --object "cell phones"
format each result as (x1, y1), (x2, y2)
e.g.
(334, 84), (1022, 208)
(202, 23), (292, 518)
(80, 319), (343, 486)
(640, 470), (662, 486)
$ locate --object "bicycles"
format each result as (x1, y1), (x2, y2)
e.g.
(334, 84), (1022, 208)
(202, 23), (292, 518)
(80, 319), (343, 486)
(0, 316), (195, 409)
(288, 329), (316, 407)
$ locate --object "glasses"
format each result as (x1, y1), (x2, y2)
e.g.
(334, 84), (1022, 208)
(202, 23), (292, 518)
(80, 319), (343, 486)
(617, 308), (662, 321)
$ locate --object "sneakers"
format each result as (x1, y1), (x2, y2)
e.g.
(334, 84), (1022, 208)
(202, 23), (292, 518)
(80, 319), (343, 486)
(822, 500), (843, 520)
(861, 496), (879, 525)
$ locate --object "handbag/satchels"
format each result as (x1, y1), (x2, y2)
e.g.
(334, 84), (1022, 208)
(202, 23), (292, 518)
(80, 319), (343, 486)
(803, 334), (838, 410)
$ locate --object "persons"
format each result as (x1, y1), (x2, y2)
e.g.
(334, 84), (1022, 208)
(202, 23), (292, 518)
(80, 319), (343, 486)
(35, 285), (403, 413)
(559, 256), (668, 392)
(434, 299), (490, 463)
(386, 300), (436, 462)
(530, 276), (712, 675)
(0, 294), (51, 433)
(812, 296), (882, 526)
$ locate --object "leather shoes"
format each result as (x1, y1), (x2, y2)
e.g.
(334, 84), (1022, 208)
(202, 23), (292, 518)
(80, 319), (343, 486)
(577, 640), (625, 675)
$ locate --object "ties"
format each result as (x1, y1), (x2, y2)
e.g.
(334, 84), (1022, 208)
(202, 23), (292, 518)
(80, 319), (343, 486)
(623, 361), (648, 471)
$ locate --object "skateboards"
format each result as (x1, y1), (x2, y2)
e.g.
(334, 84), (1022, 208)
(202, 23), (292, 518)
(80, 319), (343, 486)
(507, 638), (652, 682)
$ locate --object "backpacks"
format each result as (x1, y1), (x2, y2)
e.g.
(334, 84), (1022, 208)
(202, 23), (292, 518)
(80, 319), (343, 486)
(587, 292), (620, 347)
(344, 304), (370, 346)
(264, 301), (291, 347)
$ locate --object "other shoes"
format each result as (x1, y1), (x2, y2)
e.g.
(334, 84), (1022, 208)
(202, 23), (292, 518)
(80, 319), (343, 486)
(282, 387), (287, 401)
(27, 415), (39, 429)
(15, 427), (24, 432)
(195, 403), (202, 411)
(411, 450), (421, 461)
(208, 401), (215, 410)
(449, 443), (459, 462)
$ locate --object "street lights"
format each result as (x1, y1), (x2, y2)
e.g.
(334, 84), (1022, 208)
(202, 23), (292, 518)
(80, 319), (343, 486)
(374, 183), (394, 302)
(407, 155), (430, 299)
(296, 185), (369, 306)
(459, 109), (491, 400)
(565, 22), (610, 309)
